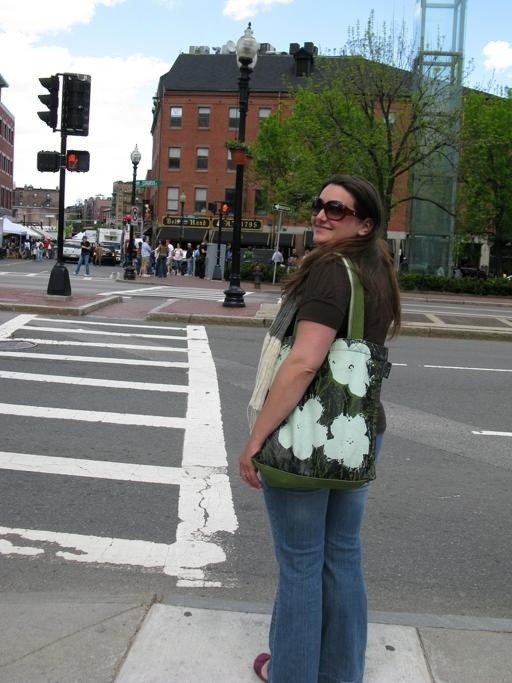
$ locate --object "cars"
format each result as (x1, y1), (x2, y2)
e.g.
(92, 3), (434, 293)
(63, 227), (123, 265)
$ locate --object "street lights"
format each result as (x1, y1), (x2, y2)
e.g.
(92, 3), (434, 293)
(123, 143), (141, 280)
(179, 191), (186, 243)
(222, 21), (258, 309)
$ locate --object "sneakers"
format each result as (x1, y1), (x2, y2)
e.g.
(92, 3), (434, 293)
(72, 272), (90, 276)
(138, 269), (193, 278)
(253, 654), (271, 682)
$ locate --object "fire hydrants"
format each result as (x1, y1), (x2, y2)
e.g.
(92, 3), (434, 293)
(252, 265), (264, 289)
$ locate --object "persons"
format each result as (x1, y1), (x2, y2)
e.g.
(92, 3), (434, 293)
(71, 236), (92, 277)
(271, 249), (285, 265)
(455, 264), (489, 280)
(92, 243), (103, 266)
(226, 243), (233, 264)
(242, 245), (257, 262)
(301, 244), (311, 260)
(286, 248), (298, 276)
(121, 235), (207, 279)
(401, 259), (410, 273)
(2, 236), (55, 262)
(239, 174), (403, 682)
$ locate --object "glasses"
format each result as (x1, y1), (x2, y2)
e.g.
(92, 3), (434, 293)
(310, 197), (361, 220)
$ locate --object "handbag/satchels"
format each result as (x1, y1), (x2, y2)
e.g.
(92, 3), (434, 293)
(253, 251), (391, 491)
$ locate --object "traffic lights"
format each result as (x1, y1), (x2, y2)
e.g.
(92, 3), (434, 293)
(38, 76), (58, 128)
(66, 150), (89, 172)
(208, 202), (217, 212)
(221, 202), (230, 212)
